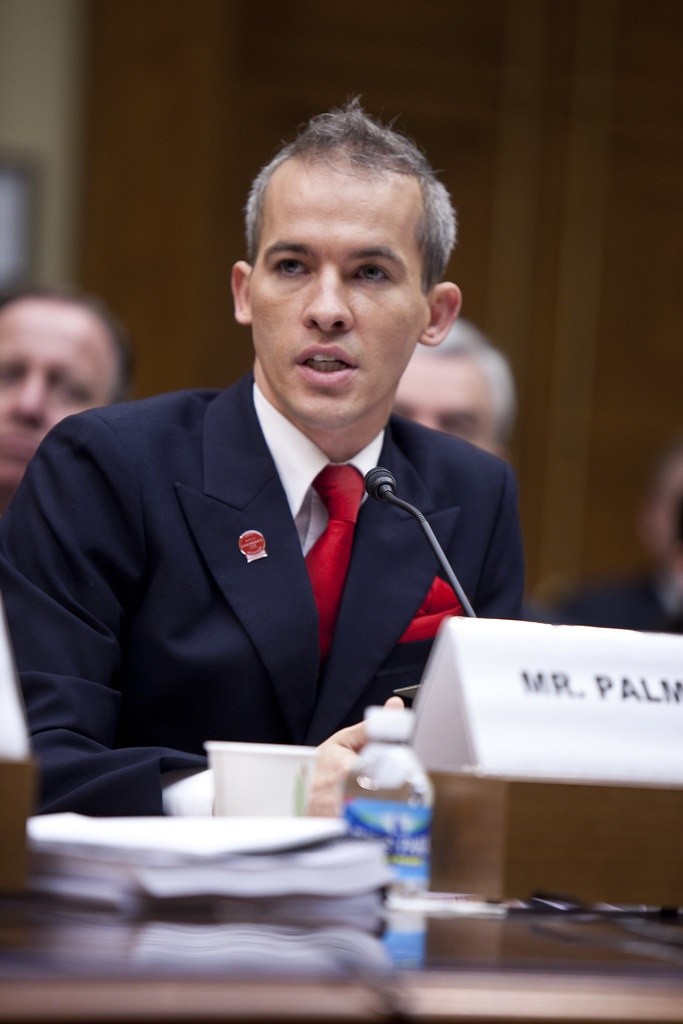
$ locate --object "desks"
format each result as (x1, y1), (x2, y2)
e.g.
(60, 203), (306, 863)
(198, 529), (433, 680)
(0, 894), (683, 1024)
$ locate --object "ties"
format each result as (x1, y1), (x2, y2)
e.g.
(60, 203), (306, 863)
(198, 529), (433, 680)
(303, 464), (362, 659)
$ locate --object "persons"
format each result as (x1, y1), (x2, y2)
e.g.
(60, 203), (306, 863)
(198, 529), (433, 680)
(0, 292), (131, 514)
(0, 110), (525, 819)
(390, 321), (516, 462)
(546, 465), (683, 631)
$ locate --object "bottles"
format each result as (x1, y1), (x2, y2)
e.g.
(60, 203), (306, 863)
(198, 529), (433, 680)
(341, 705), (433, 968)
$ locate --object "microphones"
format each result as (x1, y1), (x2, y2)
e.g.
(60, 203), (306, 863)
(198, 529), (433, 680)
(364, 467), (478, 617)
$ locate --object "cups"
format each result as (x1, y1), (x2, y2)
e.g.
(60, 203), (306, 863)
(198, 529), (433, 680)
(204, 736), (316, 824)
